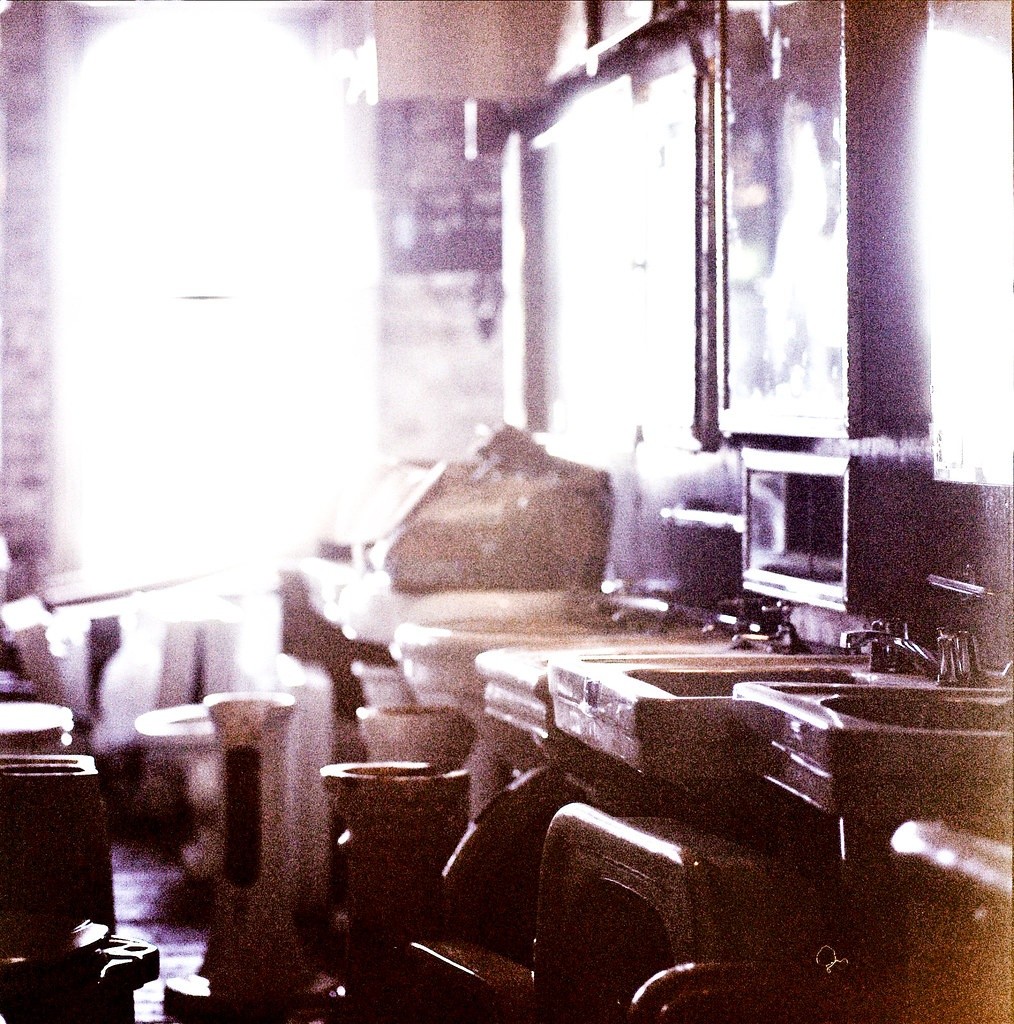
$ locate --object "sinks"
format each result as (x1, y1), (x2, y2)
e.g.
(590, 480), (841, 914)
(736, 678), (1014, 817)
(623, 663), (869, 703)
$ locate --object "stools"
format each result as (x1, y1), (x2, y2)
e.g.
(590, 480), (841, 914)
(530, 803), (832, 1024)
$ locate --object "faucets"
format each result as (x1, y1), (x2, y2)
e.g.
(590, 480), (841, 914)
(839, 627), (982, 687)
(731, 601), (812, 655)
